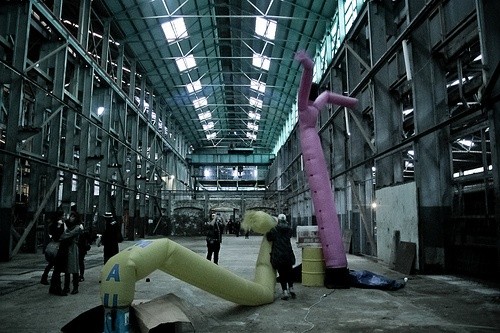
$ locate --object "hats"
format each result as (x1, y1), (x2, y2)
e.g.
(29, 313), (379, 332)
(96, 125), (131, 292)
(278, 213), (289, 224)
(209, 213), (215, 218)
(102, 211), (114, 218)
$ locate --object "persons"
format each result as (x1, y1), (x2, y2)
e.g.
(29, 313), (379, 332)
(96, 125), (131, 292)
(266, 214), (297, 300)
(39, 211), (86, 296)
(98, 212), (124, 283)
(227, 221), (241, 237)
(204, 214), (221, 265)
(215, 213), (224, 243)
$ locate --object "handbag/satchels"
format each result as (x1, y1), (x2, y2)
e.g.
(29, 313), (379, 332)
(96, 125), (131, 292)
(45, 241), (60, 256)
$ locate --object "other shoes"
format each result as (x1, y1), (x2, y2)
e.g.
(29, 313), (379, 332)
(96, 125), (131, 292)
(281, 296), (290, 301)
(49, 288), (68, 296)
(62, 288), (70, 293)
(40, 276), (49, 285)
(290, 291), (296, 299)
(71, 283), (79, 294)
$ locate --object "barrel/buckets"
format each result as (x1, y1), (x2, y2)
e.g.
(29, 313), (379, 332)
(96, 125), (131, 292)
(301, 248), (325, 287)
